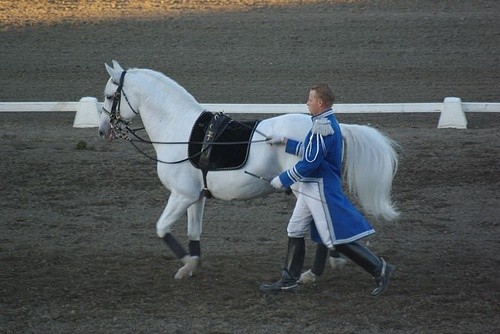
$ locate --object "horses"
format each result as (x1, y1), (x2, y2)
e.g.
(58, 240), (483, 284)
(98, 58), (403, 281)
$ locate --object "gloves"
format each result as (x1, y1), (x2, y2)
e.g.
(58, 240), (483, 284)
(270, 176), (283, 189)
(265, 135), (285, 144)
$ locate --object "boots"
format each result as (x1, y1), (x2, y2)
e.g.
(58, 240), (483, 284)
(333, 241), (397, 297)
(260, 237), (305, 291)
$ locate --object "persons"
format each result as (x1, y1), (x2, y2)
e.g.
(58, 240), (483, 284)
(259, 82), (396, 298)
